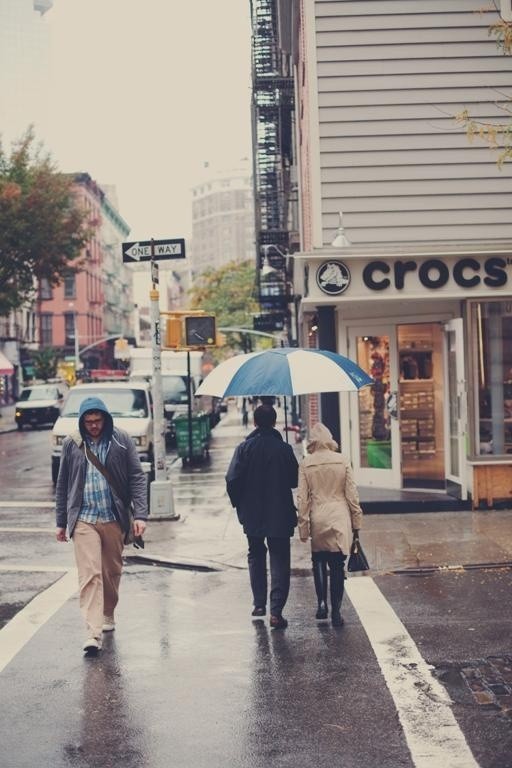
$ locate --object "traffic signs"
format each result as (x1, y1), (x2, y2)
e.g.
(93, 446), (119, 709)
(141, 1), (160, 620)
(120, 238), (186, 263)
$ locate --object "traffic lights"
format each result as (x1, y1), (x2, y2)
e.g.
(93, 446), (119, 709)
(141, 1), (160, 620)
(164, 317), (182, 347)
(179, 314), (220, 351)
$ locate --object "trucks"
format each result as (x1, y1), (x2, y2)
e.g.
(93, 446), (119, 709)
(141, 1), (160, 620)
(128, 348), (222, 445)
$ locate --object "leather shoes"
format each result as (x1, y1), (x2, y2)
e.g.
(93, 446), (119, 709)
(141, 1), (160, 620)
(251, 606), (266, 616)
(269, 616), (288, 627)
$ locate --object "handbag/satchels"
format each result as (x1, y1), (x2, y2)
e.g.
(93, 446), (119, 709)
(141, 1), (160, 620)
(348, 551), (369, 572)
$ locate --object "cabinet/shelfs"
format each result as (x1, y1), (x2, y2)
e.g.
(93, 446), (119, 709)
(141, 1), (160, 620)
(478, 388), (511, 455)
(357, 376), (436, 461)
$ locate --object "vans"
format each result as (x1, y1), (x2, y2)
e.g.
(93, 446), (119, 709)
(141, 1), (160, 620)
(15, 382), (69, 429)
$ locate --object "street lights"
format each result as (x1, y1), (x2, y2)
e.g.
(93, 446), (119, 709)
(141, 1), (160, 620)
(67, 302), (81, 372)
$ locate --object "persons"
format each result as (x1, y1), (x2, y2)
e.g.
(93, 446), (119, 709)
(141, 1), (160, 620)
(297, 421), (364, 628)
(247, 395), (260, 412)
(258, 396), (278, 410)
(225, 403), (301, 629)
(54, 395), (149, 655)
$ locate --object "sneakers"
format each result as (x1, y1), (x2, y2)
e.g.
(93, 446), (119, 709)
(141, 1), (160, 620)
(102, 614), (114, 632)
(83, 639), (102, 651)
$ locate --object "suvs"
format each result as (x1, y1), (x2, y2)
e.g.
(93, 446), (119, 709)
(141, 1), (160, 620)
(48, 376), (168, 488)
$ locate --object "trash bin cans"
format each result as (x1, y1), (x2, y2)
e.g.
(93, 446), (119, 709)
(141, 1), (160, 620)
(171, 411), (211, 465)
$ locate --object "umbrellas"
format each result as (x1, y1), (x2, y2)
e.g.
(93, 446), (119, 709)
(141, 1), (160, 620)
(191, 344), (377, 444)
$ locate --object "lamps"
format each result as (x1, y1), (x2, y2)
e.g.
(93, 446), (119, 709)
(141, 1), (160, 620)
(330, 207), (351, 249)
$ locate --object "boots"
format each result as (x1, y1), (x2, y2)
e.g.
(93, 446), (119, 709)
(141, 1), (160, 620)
(313, 559), (328, 619)
(329, 568), (344, 626)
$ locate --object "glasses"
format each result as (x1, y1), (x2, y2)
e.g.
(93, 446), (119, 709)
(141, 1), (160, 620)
(82, 417), (104, 426)
